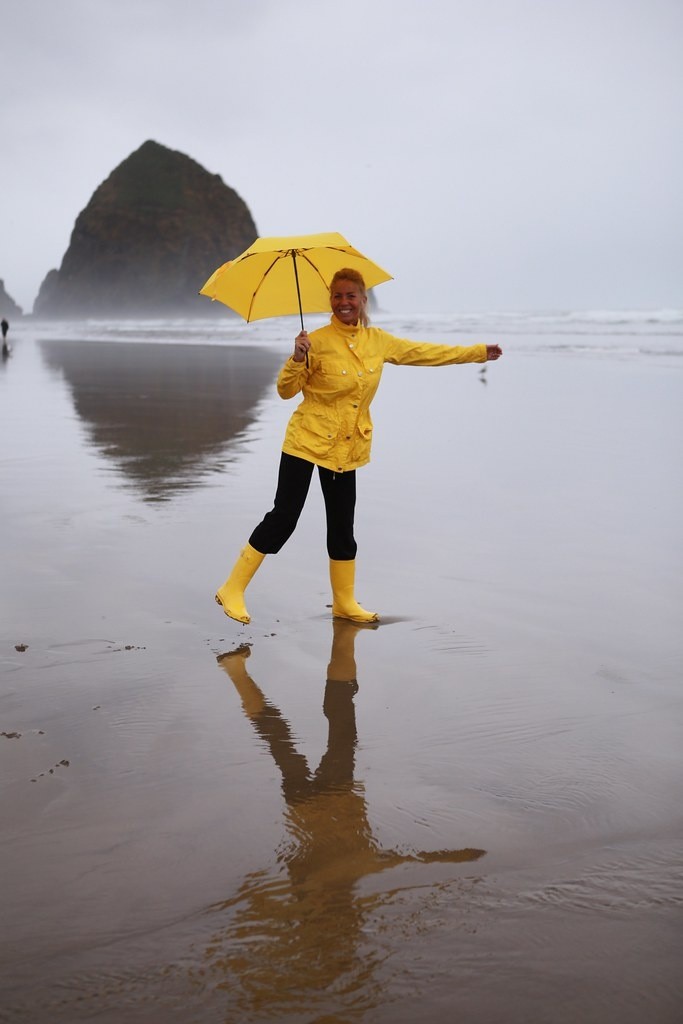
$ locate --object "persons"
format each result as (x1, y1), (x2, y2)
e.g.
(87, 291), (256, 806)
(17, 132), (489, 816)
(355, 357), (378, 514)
(212, 267), (503, 626)
(0, 317), (10, 340)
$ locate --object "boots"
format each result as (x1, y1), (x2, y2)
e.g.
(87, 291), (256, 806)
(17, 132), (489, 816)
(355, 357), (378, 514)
(329, 558), (380, 622)
(327, 616), (380, 681)
(215, 540), (267, 625)
(217, 647), (267, 717)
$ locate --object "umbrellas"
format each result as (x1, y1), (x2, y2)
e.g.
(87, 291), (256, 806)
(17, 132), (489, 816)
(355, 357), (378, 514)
(197, 229), (394, 370)
